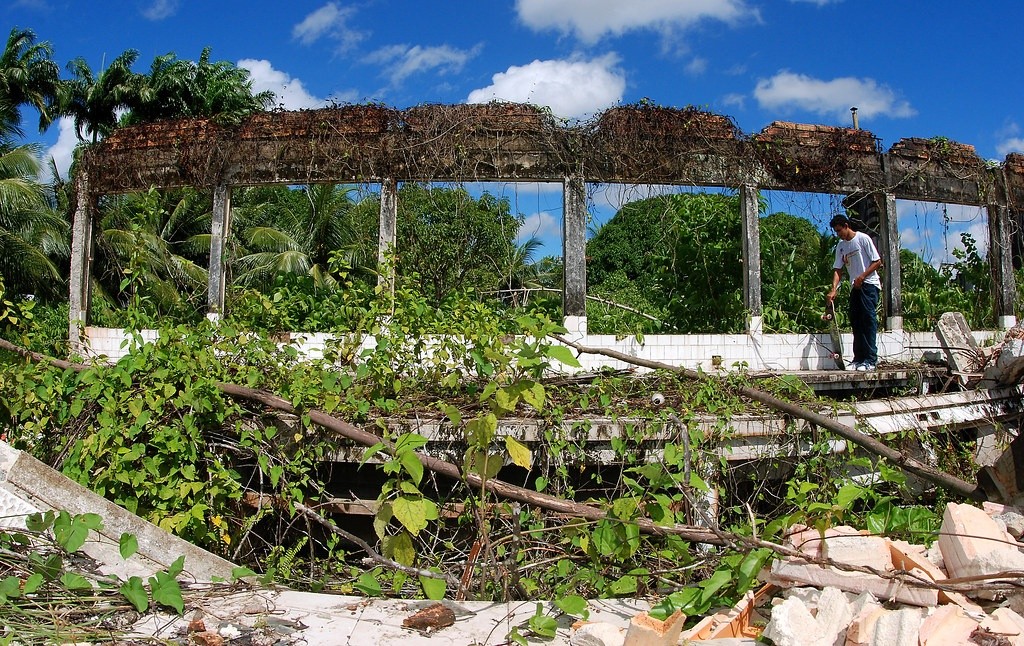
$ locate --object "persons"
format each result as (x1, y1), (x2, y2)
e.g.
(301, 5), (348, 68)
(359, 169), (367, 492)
(827, 215), (883, 373)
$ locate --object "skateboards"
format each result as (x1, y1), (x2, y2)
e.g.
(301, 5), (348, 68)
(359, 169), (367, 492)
(821, 300), (846, 370)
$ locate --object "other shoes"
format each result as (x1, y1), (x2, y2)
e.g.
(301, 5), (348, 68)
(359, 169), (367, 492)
(845, 362), (859, 371)
(857, 363), (875, 371)
